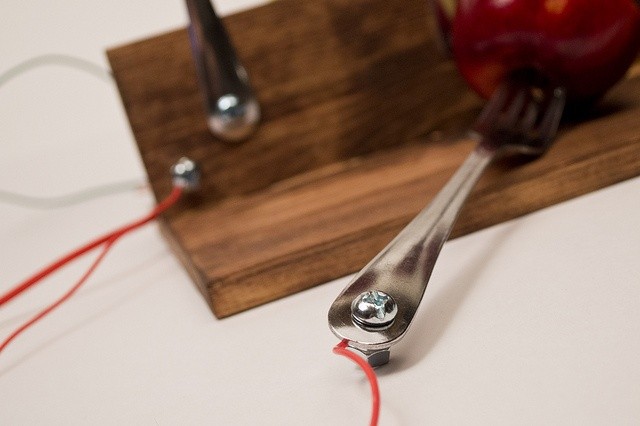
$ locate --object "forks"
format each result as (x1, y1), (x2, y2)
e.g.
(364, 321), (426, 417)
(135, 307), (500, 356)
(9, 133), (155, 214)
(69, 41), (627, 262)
(328, 81), (564, 350)
